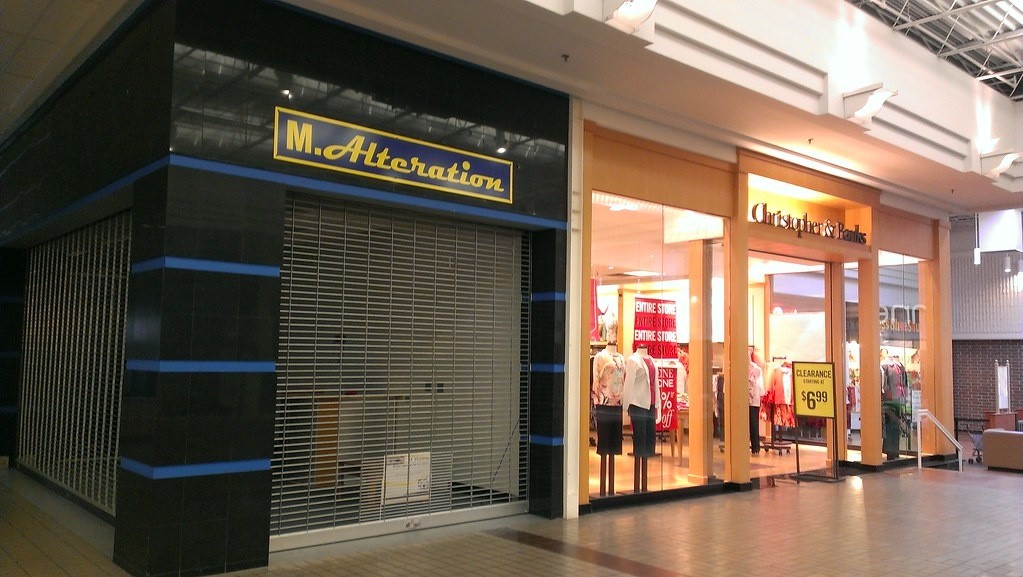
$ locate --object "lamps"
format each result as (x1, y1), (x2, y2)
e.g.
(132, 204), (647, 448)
(1003, 252), (1012, 273)
(1018, 253), (1023, 273)
(973, 213), (982, 265)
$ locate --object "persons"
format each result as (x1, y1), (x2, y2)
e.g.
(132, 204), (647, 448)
(623, 346), (660, 493)
(749, 362), (763, 453)
(881, 356), (908, 461)
(593, 343), (626, 497)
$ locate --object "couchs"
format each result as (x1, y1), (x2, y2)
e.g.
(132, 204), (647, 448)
(983, 427), (1023, 474)
